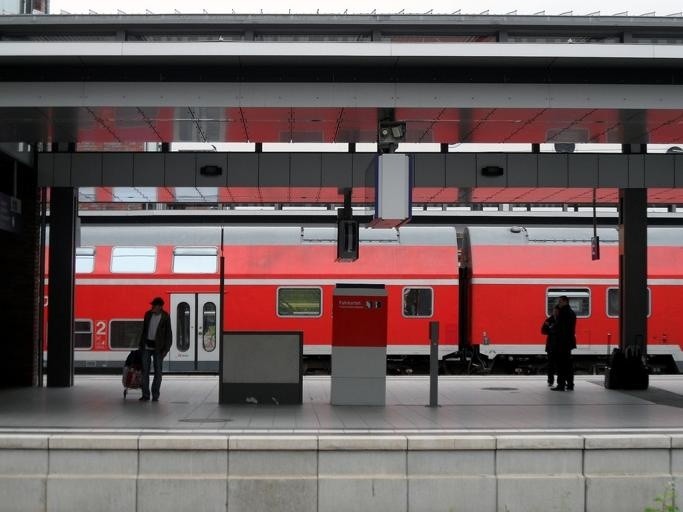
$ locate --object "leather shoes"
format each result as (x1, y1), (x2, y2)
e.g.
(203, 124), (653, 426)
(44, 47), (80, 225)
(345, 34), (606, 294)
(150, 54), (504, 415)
(139, 396), (150, 401)
(152, 393), (160, 401)
(548, 383), (573, 391)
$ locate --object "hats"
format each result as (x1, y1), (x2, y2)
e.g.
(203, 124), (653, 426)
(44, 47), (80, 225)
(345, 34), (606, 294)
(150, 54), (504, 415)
(149, 298), (164, 306)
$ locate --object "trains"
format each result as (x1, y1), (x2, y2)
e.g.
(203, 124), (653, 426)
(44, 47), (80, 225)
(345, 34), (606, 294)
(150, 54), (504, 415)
(39, 218), (682, 378)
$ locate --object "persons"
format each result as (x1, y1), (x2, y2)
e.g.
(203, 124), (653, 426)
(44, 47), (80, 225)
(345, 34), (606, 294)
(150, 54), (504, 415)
(541, 296), (577, 391)
(138, 297), (172, 401)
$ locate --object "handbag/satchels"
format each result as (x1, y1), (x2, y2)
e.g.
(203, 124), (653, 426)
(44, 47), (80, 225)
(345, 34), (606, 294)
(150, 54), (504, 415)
(124, 350), (143, 369)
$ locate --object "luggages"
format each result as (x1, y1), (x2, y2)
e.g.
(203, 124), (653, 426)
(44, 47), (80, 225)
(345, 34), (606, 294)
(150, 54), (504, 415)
(122, 350), (143, 388)
